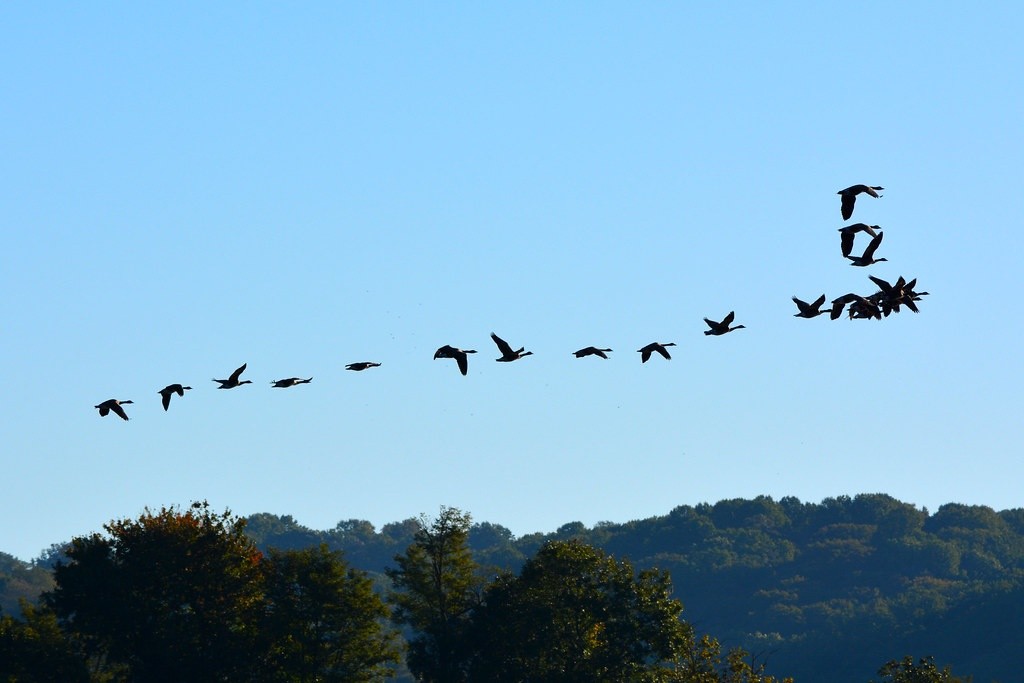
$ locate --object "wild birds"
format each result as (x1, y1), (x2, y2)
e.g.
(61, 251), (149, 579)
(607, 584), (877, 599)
(94, 184), (930, 421)
